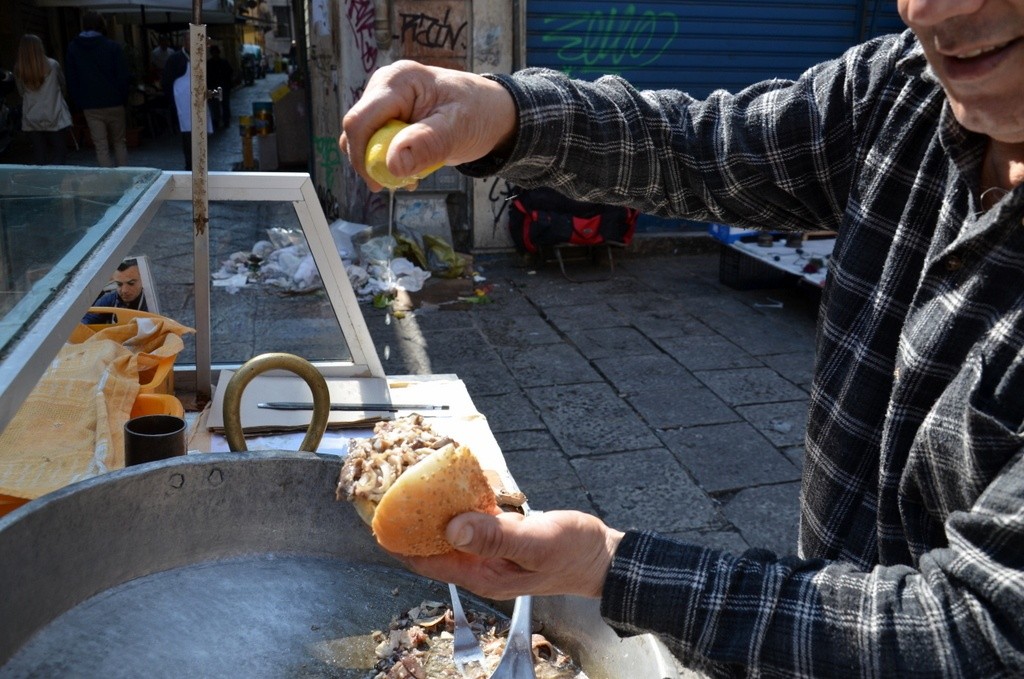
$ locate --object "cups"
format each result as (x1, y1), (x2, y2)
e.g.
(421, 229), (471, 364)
(124, 416), (189, 468)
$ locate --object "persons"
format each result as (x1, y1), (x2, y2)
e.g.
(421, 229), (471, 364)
(14, 34), (81, 167)
(150, 31), (231, 170)
(336, 0), (1024, 679)
(63, 11), (134, 167)
(80, 259), (149, 324)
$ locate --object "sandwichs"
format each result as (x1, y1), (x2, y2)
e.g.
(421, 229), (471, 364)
(333, 414), (500, 557)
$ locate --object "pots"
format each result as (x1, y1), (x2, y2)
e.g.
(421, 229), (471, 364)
(0, 351), (682, 679)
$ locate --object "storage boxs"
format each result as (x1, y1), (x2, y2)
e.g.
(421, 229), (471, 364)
(0, 304), (187, 518)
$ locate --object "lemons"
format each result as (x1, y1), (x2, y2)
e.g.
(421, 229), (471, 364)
(365, 119), (444, 189)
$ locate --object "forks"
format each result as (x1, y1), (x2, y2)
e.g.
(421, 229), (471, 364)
(447, 582), (490, 679)
